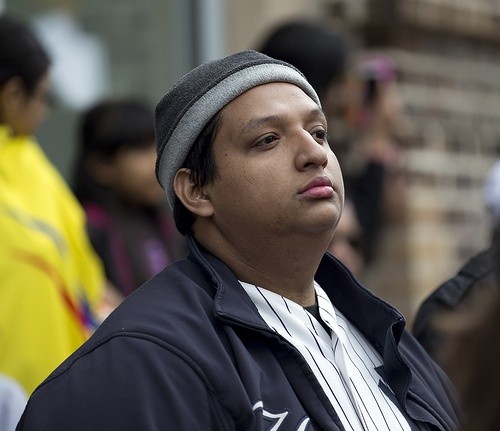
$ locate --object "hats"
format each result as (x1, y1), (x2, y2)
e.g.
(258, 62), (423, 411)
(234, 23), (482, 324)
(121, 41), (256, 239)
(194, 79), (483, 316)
(153, 49), (322, 210)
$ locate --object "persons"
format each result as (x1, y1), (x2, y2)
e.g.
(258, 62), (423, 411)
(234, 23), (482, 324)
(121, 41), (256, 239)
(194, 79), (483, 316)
(0, 16), (125, 395)
(258, 23), (460, 322)
(413, 161), (500, 431)
(15, 50), (466, 431)
(69, 99), (188, 295)
